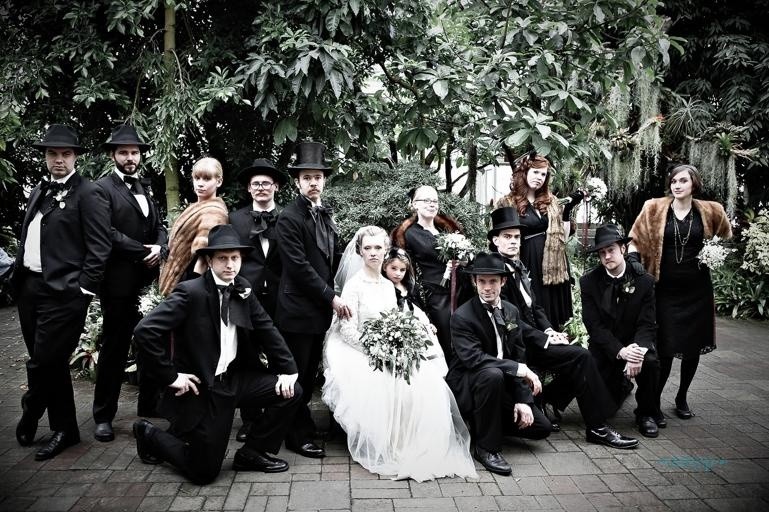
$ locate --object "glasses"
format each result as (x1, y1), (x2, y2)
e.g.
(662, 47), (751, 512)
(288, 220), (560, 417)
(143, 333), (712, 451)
(249, 181), (275, 190)
(415, 198), (441, 205)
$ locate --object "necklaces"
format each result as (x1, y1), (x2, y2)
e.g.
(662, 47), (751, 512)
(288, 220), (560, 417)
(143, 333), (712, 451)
(671, 206), (694, 264)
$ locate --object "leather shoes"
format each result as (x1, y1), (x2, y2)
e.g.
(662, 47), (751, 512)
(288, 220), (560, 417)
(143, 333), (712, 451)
(94, 422), (114, 442)
(35, 421), (80, 460)
(16, 394), (38, 447)
(232, 423), (325, 473)
(586, 423), (638, 449)
(133, 418), (164, 464)
(652, 410), (666, 428)
(538, 403), (561, 432)
(638, 416), (658, 437)
(675, 398), (691, 419)
(474, 444), (512, 474)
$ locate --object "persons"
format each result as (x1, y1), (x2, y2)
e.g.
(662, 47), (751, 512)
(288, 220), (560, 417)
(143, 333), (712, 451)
(13, 123), (112, 461)
(92, 124), (168, 443)
(131, 141), (659, 485)
(627, 165), (733, 428)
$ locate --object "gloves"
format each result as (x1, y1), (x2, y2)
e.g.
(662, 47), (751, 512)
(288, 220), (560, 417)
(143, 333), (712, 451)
(627, 255), (647, 277)
(563, 190), (584, 221)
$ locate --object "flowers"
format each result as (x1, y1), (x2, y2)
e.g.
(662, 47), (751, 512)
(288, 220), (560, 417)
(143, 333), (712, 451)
(696, 234), (738, 271)
(434, 229), (475, 286)
(557, 177), (607, 207)
(359, 308), (439, 386)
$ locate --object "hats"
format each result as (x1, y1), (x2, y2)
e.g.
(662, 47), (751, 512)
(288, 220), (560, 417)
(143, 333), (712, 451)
(486, 206), (529, 242)
(195, 224), (256, 258)
(384, 246), (411, 263)
(31, 124), (90, 155)
(461, 251), (515, 278)
(285, 141), (334, 179)
(238, 158), (287, 186)
(586, 224), (633, 254)
(102, 125), (151, 153)
